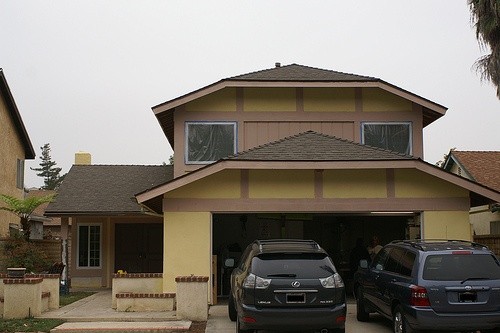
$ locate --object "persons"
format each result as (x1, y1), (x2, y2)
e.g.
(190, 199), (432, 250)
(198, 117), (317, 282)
(349, 238), (372, 295)
(365, 233), (383, 263)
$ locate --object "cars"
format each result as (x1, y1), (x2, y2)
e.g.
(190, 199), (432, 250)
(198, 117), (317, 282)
(226, 238), (347, 333)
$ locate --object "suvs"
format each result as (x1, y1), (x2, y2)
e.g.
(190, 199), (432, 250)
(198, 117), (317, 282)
(354, 239), (499, 333)
